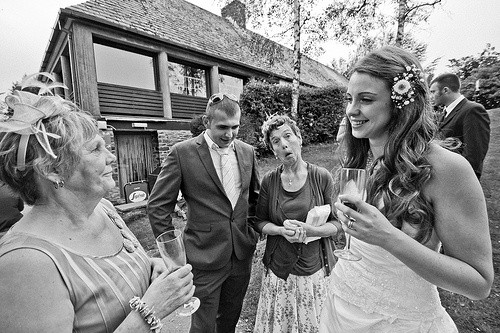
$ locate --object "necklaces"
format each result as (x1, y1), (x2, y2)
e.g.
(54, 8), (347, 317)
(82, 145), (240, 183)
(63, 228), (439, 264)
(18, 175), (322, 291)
(285, 162), (305, 186)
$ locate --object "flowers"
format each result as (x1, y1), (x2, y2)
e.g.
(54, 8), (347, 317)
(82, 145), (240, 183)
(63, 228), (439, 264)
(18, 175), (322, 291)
(0, 72), (70, 171)
(391, 64), (424, 109)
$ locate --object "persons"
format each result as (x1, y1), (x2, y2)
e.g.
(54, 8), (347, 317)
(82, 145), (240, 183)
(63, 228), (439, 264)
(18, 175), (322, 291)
(0, 190), (24, 231)
(147, 93), (260, 333)
(430, 73), (491, 180)
(320, 46), (494, 333)
(252, 111), (342, 333)
(189, 115), (207, 138)
(0, 72), (195, 333)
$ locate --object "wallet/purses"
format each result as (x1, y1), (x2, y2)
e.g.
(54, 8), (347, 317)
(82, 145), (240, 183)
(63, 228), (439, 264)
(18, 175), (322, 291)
(304, 205), (331, 244)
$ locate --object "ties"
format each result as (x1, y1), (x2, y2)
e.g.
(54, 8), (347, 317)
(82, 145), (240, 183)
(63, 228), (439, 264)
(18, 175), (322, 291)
(437, 113), (447, 129)
(216, 148), (237, 210)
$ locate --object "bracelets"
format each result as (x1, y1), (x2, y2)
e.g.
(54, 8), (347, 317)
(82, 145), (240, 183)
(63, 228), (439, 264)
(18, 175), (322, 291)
(128, 296), (163, 333)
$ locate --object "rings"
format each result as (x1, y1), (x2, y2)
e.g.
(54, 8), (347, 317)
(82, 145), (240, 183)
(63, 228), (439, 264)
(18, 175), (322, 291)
(348, 220), (353, 228)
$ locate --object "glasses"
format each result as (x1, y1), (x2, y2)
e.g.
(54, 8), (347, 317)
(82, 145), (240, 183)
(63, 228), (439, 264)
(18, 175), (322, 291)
(209, 93), (241, 106)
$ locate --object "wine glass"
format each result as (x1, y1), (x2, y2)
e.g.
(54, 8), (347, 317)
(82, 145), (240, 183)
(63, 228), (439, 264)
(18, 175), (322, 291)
(156, 230), (201, 316)
(333, 168), (366, 262)
